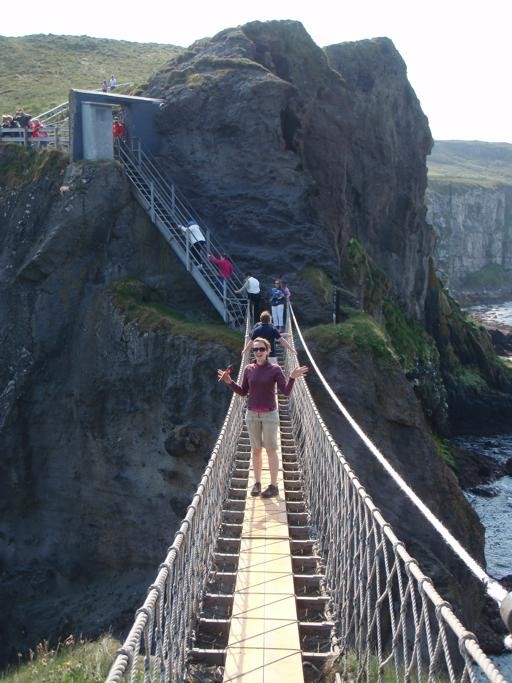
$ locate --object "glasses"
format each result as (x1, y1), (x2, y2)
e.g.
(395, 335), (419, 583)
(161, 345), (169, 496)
(251, 348), (266, 352)
(274, 283), (279, 284)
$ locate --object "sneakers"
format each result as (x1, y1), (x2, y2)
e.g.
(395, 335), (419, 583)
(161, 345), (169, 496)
(261, 485), (279, 498)
(197, 263), (203, 270)
(251, 483), (261, 496)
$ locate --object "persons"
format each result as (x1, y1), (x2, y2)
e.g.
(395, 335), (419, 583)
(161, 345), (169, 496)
(207, 253), (233, 302)
(217, 337), (309, 498)
(0, 108), (49, 149)
(109, 75), (116, 91)
(234, 273), (297, 363)
(178, 221), (206, 275)
(102, 80), (108, 93)
(112, 117), (129, 164)
